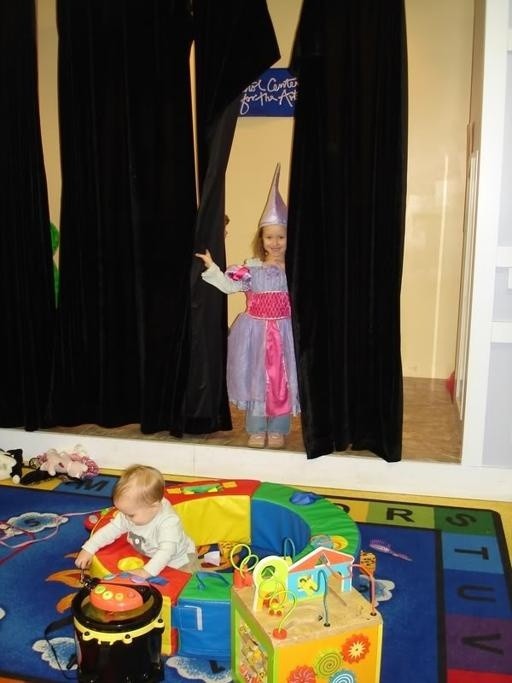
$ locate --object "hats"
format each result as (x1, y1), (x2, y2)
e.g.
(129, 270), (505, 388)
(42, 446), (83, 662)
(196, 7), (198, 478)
(257, 162), (288, 228)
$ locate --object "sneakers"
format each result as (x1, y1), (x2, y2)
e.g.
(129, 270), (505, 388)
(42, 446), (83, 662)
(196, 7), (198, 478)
(247, 432), (284, 449)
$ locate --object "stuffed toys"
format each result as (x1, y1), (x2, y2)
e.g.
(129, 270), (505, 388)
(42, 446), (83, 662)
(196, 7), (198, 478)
(0, 444), (100, 484)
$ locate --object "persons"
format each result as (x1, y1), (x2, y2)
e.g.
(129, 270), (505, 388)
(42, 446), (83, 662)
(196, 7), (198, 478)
(73, 463), (196, 581)
(194, 224), (300, 448)
